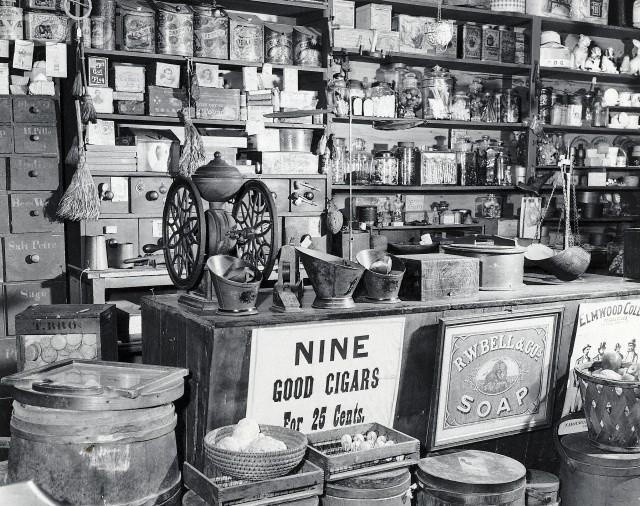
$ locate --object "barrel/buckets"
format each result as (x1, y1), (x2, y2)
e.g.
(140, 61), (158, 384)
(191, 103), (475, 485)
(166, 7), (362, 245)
(320, 470), (414, 506)
(414, 448), (527, 504)
(527, 469), (560, 505)
(560, 432), (639, 505)
(183, 474), (320, 506)
(8, 401), (182, 505)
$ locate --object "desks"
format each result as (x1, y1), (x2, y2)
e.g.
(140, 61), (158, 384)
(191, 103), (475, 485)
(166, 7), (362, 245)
(142, 272), (640, 491)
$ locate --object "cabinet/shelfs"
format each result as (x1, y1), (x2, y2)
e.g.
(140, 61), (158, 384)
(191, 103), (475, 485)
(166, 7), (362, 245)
(329, 2), (538, 271)
(526, 2), (639, 278)
(78, 1), (332, 356)
(2, 1), (84, 445)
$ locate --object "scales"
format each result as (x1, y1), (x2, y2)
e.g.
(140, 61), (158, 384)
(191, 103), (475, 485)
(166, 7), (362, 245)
(525, 163), (592, 281)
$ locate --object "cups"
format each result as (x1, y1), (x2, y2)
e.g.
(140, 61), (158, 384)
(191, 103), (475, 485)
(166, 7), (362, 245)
(618, 90), (640, 108)
(607, 113), (639, 130)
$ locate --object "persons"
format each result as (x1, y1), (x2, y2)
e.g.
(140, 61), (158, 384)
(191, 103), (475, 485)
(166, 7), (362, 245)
(613, 342), (624, 359)
(93, 89), (104, 104)
(624, 338), (639, 363)
(161, 68), (174, 85)
(592, 341), (607, 363)
(570, 343), (592, 415)
(202, 68), (213, 84)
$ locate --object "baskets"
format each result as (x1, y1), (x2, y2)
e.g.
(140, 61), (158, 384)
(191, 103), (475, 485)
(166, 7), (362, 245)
(203, 424), (309, 480)
(574, 362), (640, 454)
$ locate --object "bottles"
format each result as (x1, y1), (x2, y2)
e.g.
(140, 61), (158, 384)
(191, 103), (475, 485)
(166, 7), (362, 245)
(329, 136), (420, 186)
(339, 81), (396, 119)
(380, 193), (405, 227)
